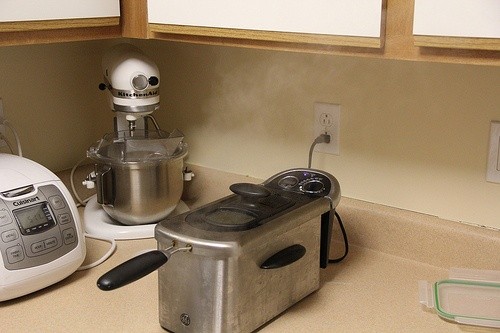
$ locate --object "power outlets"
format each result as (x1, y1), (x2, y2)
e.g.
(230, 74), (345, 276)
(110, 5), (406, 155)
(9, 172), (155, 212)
(313, 101), (341, 154)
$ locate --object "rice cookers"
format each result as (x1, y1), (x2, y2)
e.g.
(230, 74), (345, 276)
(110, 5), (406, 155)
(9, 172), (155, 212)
(0, 152), (87, 303)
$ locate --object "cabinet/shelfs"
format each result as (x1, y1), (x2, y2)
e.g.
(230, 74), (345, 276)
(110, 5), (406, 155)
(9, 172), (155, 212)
(0, 0), (499, 64)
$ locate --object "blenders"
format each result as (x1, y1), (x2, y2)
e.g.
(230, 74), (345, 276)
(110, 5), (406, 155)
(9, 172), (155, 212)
(83, 43), (192, 239)
